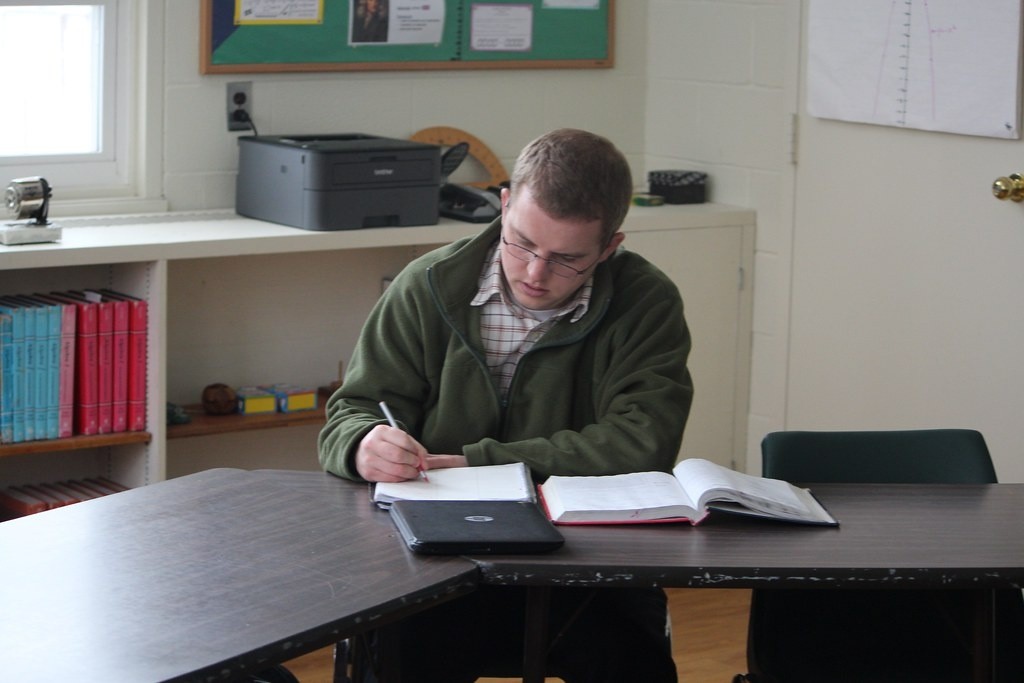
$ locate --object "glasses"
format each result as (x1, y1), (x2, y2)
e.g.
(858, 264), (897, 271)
(501, 196), (614, 281)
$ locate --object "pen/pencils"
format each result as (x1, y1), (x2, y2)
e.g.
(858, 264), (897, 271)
(378, 399), (431, 484)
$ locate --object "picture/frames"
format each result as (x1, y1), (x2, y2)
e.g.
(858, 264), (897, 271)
(198, 0), (615, 75)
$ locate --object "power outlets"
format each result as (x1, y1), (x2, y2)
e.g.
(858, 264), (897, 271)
(227, 81), (252, 131)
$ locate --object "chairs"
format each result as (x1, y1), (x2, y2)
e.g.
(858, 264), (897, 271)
(732, 429), (1024, 683)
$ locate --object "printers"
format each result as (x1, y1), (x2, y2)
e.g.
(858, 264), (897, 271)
(236, 132), (441, 231)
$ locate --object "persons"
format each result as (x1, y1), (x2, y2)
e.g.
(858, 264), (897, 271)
(353, 0), (386, 40)
(318, 127), (695, 682)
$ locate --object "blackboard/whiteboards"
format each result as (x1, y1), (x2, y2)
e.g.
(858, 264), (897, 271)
(803, 1), (1021, 140)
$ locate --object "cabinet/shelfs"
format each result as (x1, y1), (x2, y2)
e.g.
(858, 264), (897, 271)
(0, 206), (760, 485)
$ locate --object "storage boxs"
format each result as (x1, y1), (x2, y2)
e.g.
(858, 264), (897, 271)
(235, 386), (277, 415)
(647, 171), (706, 205)
(258, 382), (318, 412)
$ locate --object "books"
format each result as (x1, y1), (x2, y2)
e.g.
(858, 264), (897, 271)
(0, 474), (130, 521)
(0, 287), (148, 443)
(369, 459), (536, 508)
(386, 498), (565, 555)
(537, 458), (842, 530)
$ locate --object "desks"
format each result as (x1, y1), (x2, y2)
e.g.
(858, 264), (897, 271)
(0, 469), (1024, 683)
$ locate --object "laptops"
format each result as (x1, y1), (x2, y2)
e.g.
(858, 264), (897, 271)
(389, 499), (565, 556)
